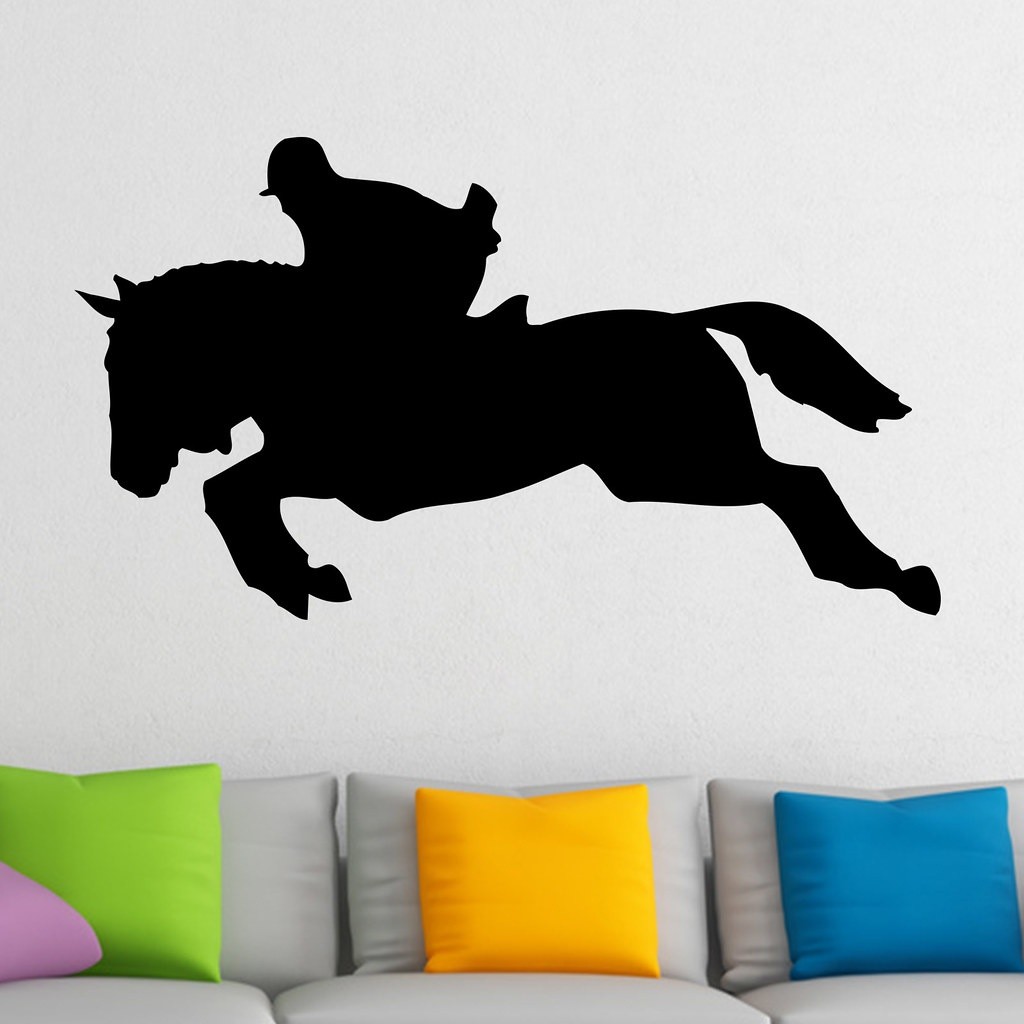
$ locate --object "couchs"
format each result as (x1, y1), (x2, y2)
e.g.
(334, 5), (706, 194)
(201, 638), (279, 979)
(0, 774), (1023, 1024)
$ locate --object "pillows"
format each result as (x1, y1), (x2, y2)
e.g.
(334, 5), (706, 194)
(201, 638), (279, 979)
(0, 861), (104, 980)
(775, 787), (1023, 980)
(416, 783), (662, 979)
(0, 763), (223, 981)
